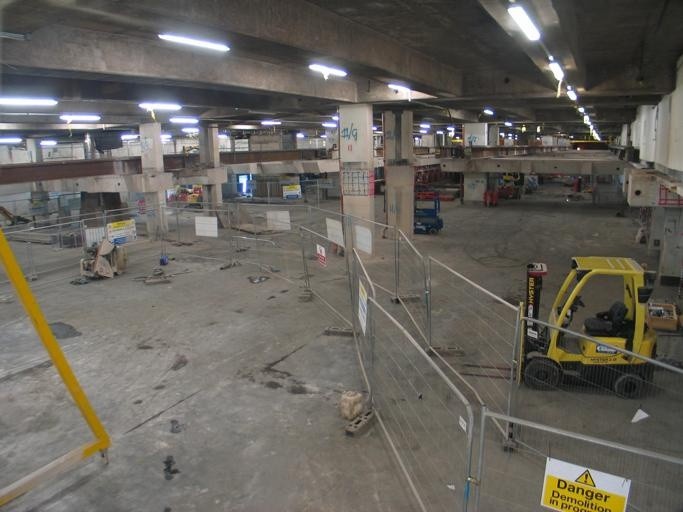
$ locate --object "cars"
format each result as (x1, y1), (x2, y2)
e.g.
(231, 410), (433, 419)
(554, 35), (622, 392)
(176, 184), (192, 194)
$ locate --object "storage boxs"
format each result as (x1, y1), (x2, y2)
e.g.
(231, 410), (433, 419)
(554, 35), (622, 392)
(648, 301), (679, 334)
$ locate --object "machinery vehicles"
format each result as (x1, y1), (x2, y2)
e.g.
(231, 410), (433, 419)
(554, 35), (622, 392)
(412, 196), (443, 236)
(455, 254), (656, 399)
(183, 184), (203, 210)
